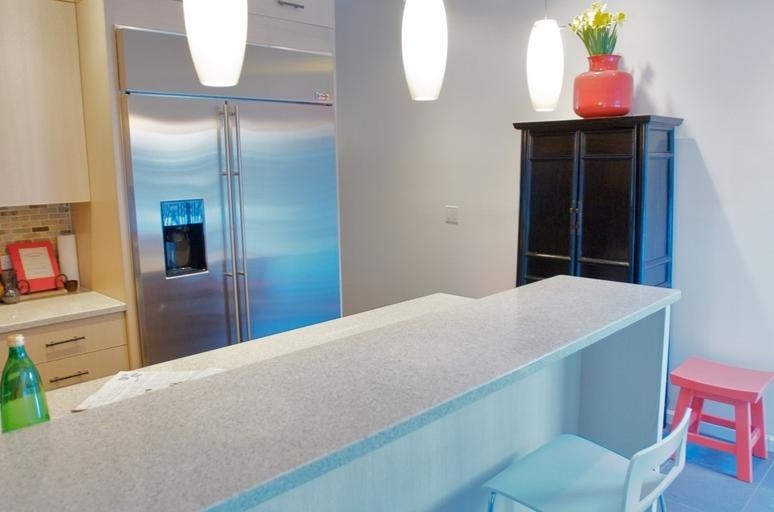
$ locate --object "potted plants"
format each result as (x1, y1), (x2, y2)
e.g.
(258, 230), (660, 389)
(7, 240), (64, 294)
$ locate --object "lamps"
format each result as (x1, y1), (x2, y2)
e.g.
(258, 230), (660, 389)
(481, 407), (692, 512)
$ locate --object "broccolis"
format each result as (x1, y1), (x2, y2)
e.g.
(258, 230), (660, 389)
(113, 20), (343, 369)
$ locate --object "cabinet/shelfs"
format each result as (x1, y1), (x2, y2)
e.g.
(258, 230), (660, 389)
(1, 334), (51, 434)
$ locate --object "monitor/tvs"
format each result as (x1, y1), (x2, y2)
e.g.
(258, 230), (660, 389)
(5, 240), (63, 295)
(70, 367), (226, 413)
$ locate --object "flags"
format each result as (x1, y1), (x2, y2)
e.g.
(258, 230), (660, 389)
(573, 54), (633, 118)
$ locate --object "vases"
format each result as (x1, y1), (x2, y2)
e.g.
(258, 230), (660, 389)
(568, 1), (627, 55)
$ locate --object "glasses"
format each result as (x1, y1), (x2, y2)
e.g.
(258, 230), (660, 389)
(526, 2), (565, 112)
(401, 0), (448, 101)
(183, 0), (248, 88)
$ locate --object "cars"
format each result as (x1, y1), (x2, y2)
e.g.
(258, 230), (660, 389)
(64, 280), (78, 293)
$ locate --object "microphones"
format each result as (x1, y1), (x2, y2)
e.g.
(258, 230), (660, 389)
(670, 355), (774, 483)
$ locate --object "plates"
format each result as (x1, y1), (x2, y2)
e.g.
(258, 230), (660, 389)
(0, 284), (130, 392)
(511, 115), (684, 427)
